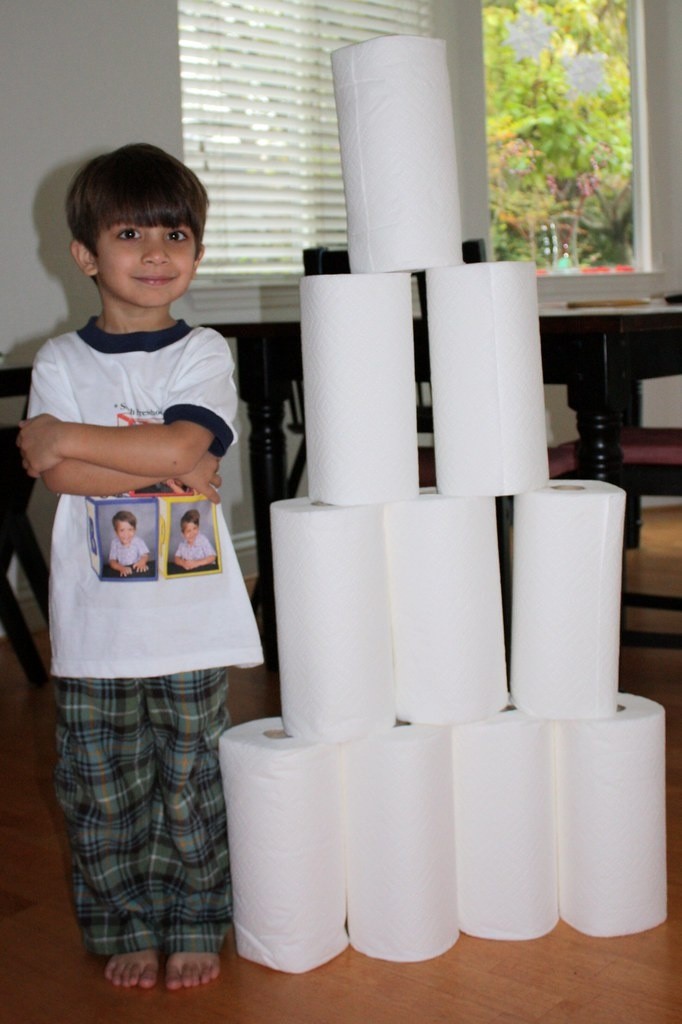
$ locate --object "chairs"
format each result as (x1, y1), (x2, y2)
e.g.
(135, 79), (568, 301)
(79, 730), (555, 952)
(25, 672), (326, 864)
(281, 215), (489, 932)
(0, 366), (53, 684)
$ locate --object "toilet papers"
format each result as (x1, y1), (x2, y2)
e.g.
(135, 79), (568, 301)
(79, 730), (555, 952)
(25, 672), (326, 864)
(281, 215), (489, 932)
(452, 691), (559, 946)
(548, 691), (671, 941)
(298, 267), (424, 512)
(329, 32), (465, 276)
(217, 712), (350, 975)
(425, 254), (555, 501)
(381, 479), (517, 732)
(505, 471), (626, 729)
(346, 711), (464, 969)
(266, 491), (393, 743)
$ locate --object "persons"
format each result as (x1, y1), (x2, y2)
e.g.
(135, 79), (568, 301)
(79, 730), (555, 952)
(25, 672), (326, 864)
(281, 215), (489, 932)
(14, 141), (269, 992)
(107, 509), (151, 578)
(172, 509), (218, 570)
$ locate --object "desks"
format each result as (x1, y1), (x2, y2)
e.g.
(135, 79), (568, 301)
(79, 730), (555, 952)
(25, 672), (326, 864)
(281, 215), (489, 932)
(533, 296), (682, 551)
